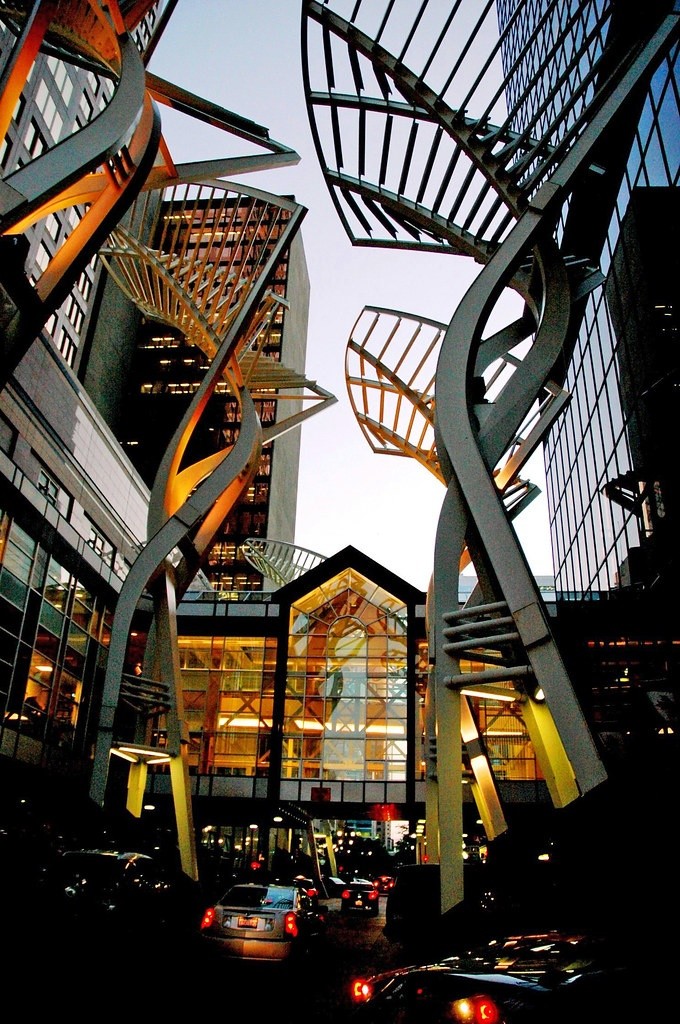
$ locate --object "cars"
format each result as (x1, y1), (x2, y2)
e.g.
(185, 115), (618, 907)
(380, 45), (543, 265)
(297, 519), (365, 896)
(269, 877), (328, 938)
(321, 875), (346, 897)
(343, 929), (680, 1024)
(199, 883), (313, 968)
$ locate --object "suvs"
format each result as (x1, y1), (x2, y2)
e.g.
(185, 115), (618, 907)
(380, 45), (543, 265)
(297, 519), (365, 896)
(342, 882), (379, 917)
